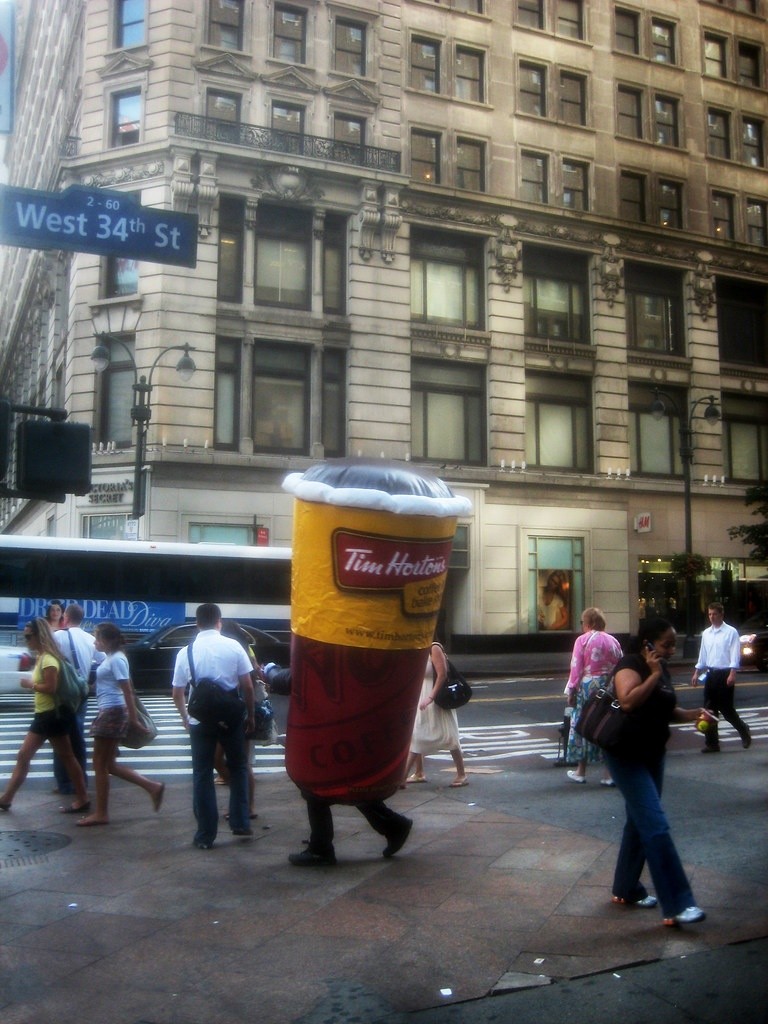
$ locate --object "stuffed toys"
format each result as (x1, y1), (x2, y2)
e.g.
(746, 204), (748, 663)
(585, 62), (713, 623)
(262, 464), (476, 866)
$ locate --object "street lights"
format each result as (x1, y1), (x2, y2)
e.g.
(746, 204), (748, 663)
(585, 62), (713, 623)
(649, 386), (722, 662)
(89, 330), (205, 546)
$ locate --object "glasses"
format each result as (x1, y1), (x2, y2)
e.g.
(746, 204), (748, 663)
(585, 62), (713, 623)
(580, 621), (584, 624)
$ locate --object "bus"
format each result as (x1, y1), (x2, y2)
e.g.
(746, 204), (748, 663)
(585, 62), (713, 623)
(0, 532), (292, 648)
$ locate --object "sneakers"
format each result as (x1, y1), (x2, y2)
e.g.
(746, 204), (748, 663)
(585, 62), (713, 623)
(662, 905), (706, 926)
(383, 815), (412, 857)
(741, 723), (752, 748)
(701, 740), (720, 753)
(611, 893), (658, 908)
(289, 849), (337, 865)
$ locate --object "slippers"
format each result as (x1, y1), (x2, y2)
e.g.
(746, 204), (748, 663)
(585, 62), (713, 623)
(153, 783), (164, 810)
(76, 816), (109, 825)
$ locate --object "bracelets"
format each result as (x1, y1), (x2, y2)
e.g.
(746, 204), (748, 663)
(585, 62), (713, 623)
(32, 683), (37, 690)
(429, 696), (434, 701)
(263, 662), (281, 678)
(179, 713), (188, 719)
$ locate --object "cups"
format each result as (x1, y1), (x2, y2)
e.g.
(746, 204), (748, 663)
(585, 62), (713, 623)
(283, 464), (473, 804)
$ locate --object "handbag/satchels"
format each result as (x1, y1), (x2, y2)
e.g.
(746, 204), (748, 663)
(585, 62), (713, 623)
(241, 701), (272, 740)
(75, 668), (90, 699)
(186, 679), (247, 740)
(574, 687), (633, 749)
(433, 659), (473, 709)
(122, 695), (158, 749)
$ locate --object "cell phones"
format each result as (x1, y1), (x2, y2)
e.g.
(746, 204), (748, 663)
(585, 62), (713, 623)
(646, 642), (655, 651)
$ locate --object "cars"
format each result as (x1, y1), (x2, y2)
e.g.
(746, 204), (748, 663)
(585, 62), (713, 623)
(86, 620), (293, 690)
(0, 645), (37, 694)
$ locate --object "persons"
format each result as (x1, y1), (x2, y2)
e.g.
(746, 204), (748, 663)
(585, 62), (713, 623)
(45, 600), (108, 793)
(75, 622), (165, 825)
(0, 618), (92, 811)
(564, 607), (623, 786)
(538, 569), (570, 630)
(604, 616), (719, 924)
(692, 602), (751, 753)
(640, 590), (677, 624)
(173, 603), (278, 849)
(399, 626), (469, 789)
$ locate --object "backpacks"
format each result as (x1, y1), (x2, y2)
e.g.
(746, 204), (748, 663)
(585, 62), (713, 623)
(58, 659), (85, 714)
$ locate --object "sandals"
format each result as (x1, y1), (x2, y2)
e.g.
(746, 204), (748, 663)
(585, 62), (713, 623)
(448, 776), (470, 787)
(59, 800), (91, 813)
(0, 803), (11, 812)
(406, 773), (425, 782)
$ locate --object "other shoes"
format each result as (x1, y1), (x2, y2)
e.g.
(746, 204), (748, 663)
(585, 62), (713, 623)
(224, 813), (230, 820)
(600, 778), (616, 787)
(233, 830), (248, 835)
(248, 813), (258, 819)
(213, 777), (226, 785)
(566, 769), (586, 783)
(197, 842), (212, 848)
